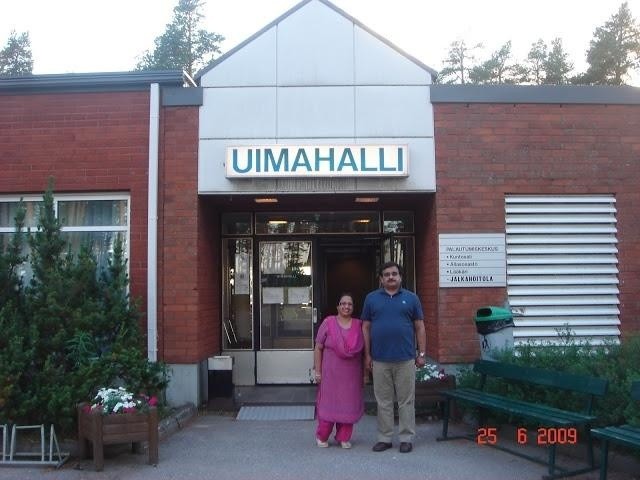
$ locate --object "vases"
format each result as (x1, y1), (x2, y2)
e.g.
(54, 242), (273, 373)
(414, 375), (457, 422)
(77, 409), (159, 471)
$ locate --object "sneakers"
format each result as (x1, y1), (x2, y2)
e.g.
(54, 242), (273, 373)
(340, 441), (352, 449)
(400, 442), (412, 453)
(316, 437), (328, 447)
(373, 441), (392, 451)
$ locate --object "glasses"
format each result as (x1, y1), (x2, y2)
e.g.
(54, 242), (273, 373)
(338, 302), (353, 307)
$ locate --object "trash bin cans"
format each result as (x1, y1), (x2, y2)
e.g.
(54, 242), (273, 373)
(474, 306), (515, 362)
(208, 356), (235, 411)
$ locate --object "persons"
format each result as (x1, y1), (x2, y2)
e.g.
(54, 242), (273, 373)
(313, 292), (365, 449)
(360, 261), (427, 453)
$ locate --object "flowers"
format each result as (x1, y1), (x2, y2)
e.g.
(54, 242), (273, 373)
(82, 384), (159, 416)
(414, 354), (448, 381)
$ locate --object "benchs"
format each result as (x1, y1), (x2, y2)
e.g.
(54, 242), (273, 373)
(435, 359), (639, 480)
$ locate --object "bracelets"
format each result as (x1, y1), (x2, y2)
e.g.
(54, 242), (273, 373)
(418, 352), (425, 358)
